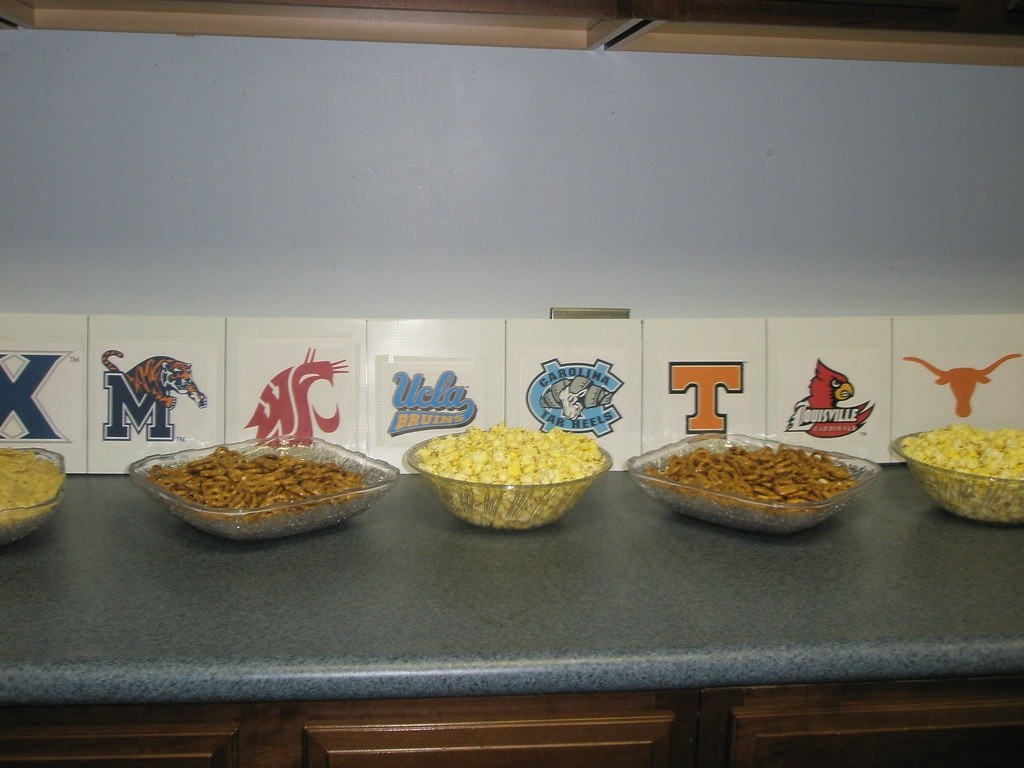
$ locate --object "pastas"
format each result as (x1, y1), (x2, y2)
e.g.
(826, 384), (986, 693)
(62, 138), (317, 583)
(145, 446), (366, 520)
(647, 444), (858, 518)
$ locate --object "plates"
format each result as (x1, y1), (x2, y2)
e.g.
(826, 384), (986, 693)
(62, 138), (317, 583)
(129, 433), (400, 541)
(626, 432), (882, 533)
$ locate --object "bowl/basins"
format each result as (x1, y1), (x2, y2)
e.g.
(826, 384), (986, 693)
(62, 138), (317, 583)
(0, 448), (66, 548)
(406, 431), (613, 531)
(892, 431), (1024, 523)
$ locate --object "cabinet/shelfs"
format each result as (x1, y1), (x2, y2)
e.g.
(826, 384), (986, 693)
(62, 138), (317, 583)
(0, 674), (1024, 768)
